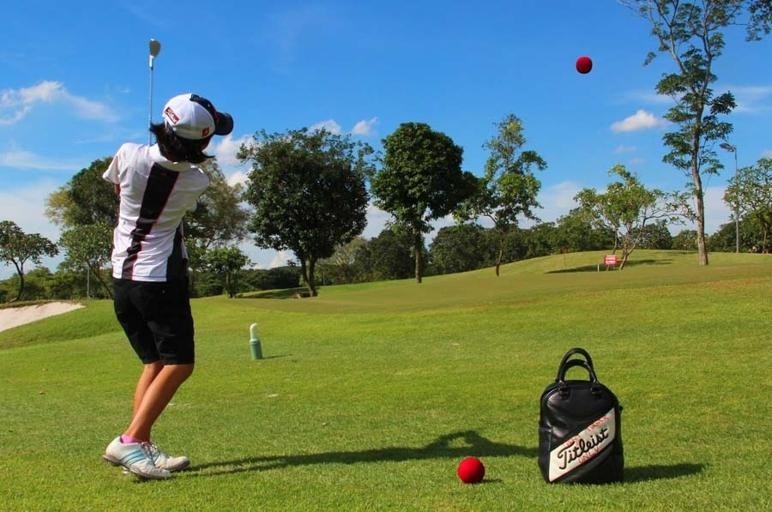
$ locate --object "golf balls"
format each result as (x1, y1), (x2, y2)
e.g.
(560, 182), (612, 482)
(576, 55), (593, 74)
(457, 456), (486, 483)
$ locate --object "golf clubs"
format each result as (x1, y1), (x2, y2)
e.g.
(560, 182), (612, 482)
(148, 37), (160, 144)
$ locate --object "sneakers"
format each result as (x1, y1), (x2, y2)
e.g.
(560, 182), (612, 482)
(102, 435), (190, 480)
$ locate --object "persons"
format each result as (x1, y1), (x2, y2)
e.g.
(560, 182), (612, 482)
(101, 93), (233, 480)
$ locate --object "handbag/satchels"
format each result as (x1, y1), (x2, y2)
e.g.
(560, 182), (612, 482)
(538, 378), (624, 485)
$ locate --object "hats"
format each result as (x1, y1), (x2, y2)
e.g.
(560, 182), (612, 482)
(161, 93), (233, 140)
